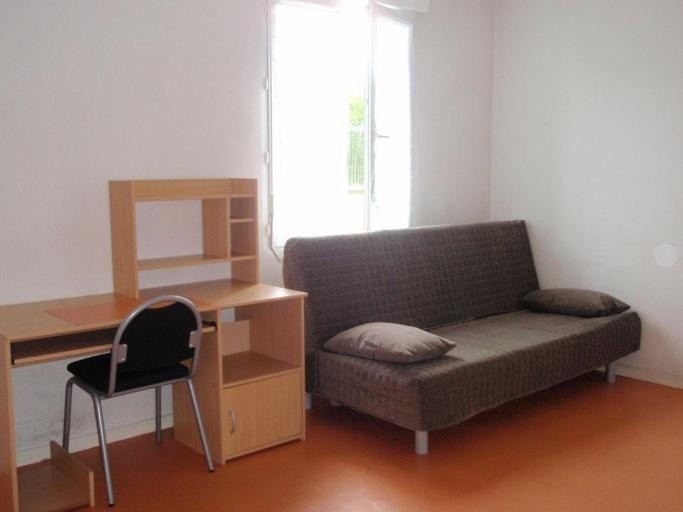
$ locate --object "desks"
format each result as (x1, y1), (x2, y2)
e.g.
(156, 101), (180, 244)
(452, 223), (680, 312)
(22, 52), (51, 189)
(0, 277), (341, 511)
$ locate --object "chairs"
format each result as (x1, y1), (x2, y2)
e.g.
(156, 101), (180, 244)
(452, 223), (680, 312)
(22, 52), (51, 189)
(60, 290), (214, 505)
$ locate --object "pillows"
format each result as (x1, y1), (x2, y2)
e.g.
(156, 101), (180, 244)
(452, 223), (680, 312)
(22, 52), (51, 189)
(326, 320), (455, 374)
(525, 283), (627, 317)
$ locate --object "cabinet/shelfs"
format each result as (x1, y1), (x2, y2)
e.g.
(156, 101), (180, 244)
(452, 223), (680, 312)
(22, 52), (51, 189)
(108, 172), (274, 295)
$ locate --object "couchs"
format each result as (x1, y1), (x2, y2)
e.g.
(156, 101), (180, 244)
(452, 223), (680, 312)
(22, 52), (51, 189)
(290, 219), (643, 457)
